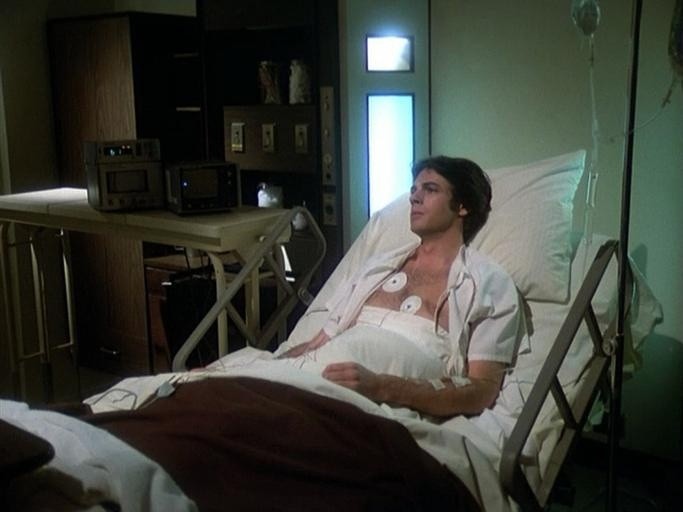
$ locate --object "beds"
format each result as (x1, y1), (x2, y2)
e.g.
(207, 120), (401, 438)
(0, 202), (634, 512)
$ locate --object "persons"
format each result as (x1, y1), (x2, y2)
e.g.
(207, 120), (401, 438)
(189, 155), (529, 423)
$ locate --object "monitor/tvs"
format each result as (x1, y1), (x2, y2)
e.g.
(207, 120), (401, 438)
(164, 161), (241, 217)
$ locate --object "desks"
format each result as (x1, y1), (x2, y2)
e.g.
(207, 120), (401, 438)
(0, 187), (293, 361)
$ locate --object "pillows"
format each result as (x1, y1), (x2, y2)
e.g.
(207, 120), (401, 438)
(311, 149), (587, 313)
